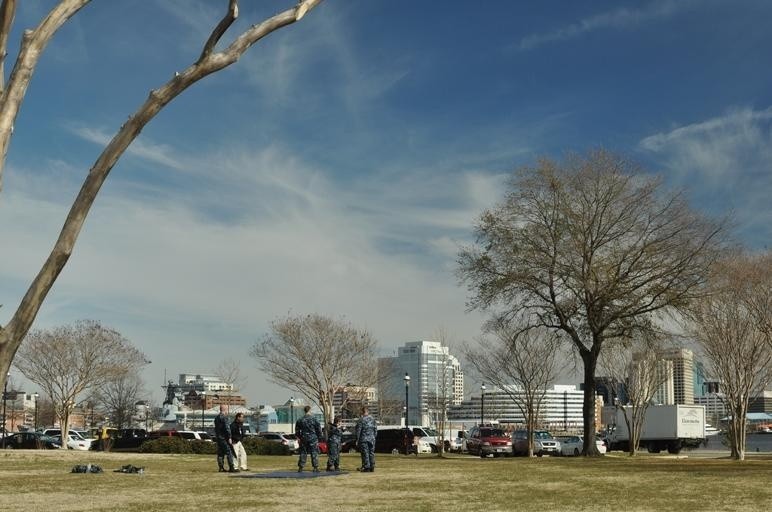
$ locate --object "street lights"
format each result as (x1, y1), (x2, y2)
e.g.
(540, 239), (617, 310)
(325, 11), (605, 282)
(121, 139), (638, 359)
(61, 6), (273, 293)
(612, 395), (620, 425)
(288, 395), (295, 433)
(32, 391), (41, 431)
(198, 389), (207, 428)
(3, 368), (11, 436)
(402, 371), (411, 428)
(144, 402), (150, 431)
(479, 381), (487, 420)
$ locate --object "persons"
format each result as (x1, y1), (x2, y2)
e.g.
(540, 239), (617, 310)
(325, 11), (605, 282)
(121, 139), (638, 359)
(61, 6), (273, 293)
(355, 406), (378, 472)
(212, 404), (238, 473)
(326, 416), (347, 472)
(295, 406), (323, 472)
(230, 412), (249, 472)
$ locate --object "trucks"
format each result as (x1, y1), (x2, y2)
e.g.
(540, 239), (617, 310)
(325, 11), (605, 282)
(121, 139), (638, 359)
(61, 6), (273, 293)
(601, 402), (710, 454)
